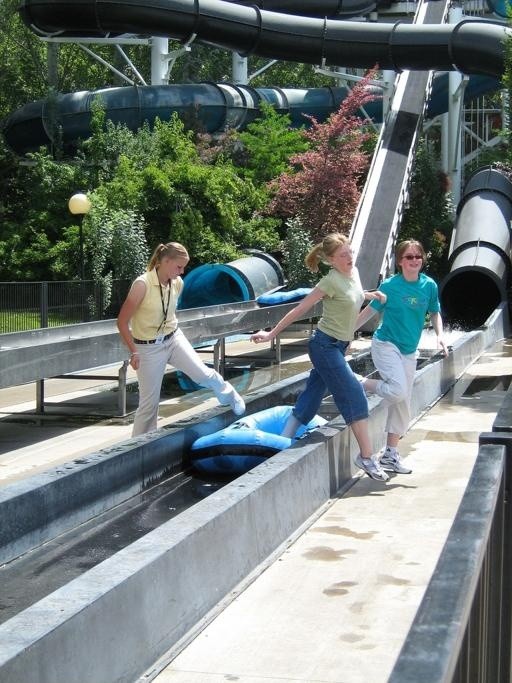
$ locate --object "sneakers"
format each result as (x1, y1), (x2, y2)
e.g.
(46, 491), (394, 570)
(230, 390), (246, 415)
(378, 452), (412, 474)
(353, 452), (390, 482)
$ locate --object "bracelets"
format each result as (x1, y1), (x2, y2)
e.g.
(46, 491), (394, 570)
(128, 351), (139, 357)
(363, 289), (367, 293)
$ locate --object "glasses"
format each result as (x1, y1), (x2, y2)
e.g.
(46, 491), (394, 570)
(330, 249), (357, 259)
(400, 254), (424, 261)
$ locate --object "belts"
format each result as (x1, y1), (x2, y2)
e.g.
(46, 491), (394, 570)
(133, 328), (179, 345)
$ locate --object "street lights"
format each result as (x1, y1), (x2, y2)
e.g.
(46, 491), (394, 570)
(68, 193), (92, 322)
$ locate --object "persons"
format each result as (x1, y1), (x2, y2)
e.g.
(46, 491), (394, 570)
(350, 235), (449, 474)
(116, 240), (246, 437)
(246, 230), (390, 483)
